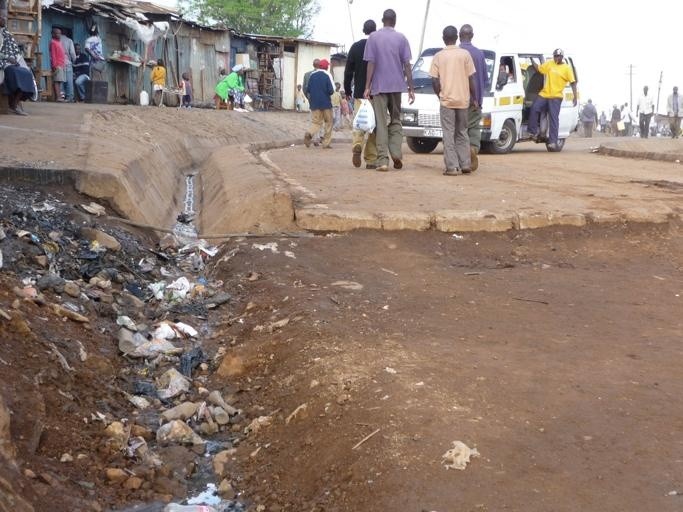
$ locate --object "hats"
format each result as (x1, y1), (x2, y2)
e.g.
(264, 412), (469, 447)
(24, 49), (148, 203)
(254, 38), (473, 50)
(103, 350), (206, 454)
(552, 48), (564, 56)
(319, 59), (330, 70)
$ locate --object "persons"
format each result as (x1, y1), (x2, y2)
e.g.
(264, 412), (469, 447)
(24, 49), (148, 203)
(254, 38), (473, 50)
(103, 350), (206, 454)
(218, 65), (227, 80)
(49, 24), (105, 102)
(214, 63), (249, 112)
(151, 58), (167, 107)
(0, 13), (38, 114)
(180, 72), (191, 108)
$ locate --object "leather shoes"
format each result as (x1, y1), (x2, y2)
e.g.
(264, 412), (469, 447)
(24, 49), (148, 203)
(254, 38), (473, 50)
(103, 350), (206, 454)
(305, 123), (353, 148)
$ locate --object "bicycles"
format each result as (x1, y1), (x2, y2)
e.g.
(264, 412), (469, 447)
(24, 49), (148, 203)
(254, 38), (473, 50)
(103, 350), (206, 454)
(17, 44), (38, 102)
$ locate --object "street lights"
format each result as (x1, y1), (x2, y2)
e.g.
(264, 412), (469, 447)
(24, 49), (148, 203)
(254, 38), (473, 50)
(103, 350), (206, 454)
(346, 0), (356, 44)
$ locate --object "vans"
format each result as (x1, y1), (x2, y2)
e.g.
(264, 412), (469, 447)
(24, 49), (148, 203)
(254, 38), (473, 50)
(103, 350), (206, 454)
(397, 45), (581, 157)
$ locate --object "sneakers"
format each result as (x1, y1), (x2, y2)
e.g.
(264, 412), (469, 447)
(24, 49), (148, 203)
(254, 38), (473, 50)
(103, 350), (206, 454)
(56, 91), (84, 103)
(443, 146), (478, 175)
(352, 145), (403, 171)
(157, 104), (191, 109)
(522, 132), (537, 138)
(7, 105), (29, 116)
(234, 107), (243, 112)
(243, 109), (249, 112)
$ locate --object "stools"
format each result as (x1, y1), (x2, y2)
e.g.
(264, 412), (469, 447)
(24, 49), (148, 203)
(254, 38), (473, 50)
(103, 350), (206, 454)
(214, 96), (231, 111)
